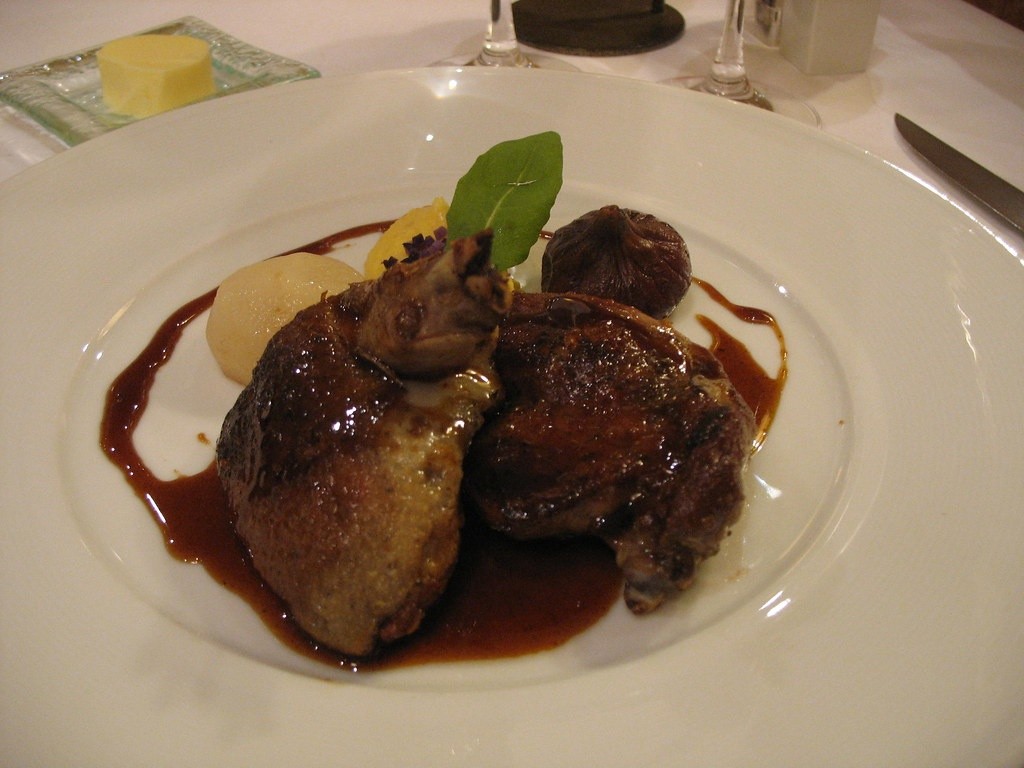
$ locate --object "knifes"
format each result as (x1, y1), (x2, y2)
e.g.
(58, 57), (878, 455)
(892, 112), (1023, 233)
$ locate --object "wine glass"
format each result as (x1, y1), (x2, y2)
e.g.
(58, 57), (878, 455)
(426, 0), (580, 76)
(659, 0), (826, 131)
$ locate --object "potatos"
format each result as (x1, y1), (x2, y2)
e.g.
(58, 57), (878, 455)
(206, 252), (366, 389)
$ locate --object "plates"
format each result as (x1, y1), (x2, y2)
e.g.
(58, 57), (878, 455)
(0, 15), (322, 150)
(1, 67), (1024, 768)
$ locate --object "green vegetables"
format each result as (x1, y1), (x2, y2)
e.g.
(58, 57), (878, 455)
(444, 130), (564, 269)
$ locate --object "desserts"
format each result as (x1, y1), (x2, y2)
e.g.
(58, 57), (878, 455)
(99, 34), (216, 119)
